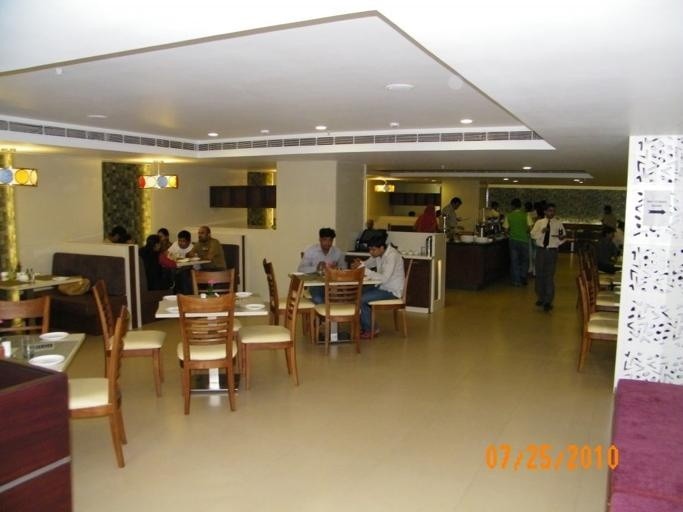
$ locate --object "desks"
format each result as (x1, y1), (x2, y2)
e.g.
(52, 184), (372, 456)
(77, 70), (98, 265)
(562, 223), (592, 252)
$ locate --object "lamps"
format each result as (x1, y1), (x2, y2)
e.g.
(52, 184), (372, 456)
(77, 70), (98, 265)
(138, 158), (181, 191)
(373, 178), (396, 193)
(0, 148), (41, 188)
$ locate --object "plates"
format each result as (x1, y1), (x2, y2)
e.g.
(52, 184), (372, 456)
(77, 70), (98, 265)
(460, 234), (493, 244)
(163, 292), (265, 313)
(28, 355), (65, 368)
(52, 277), (70, 280)
(39, 332), (68, 341)
(293, 273), (304, 276)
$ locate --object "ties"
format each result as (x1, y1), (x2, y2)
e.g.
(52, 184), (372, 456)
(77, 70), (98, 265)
(543, 220), (550, 248)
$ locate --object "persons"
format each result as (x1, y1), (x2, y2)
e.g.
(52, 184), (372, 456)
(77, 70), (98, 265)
(594, 204), (626, 276)
(102, 226), (227, 292)
(415, 196), (548, 290)
(298, 228), (350, 340)
(351, 218), (406, 339)
(529, 203), (567, 312)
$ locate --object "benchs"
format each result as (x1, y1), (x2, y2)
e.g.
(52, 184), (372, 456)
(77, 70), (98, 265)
(21, 229), (245, 335)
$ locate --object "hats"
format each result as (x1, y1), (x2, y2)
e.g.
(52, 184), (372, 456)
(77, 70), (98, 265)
(319, 228), (336, 238)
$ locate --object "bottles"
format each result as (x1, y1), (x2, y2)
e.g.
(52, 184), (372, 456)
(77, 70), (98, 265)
(316, 260), (326, 282)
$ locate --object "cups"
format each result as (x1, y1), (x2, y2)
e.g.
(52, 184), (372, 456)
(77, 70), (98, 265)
(2, 341), (12, 358)
(22, 336), (35, 359)
(1, 272), (29, 282)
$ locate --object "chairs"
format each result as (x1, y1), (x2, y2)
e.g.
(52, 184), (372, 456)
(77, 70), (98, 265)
(575, 246), (623, 373)
